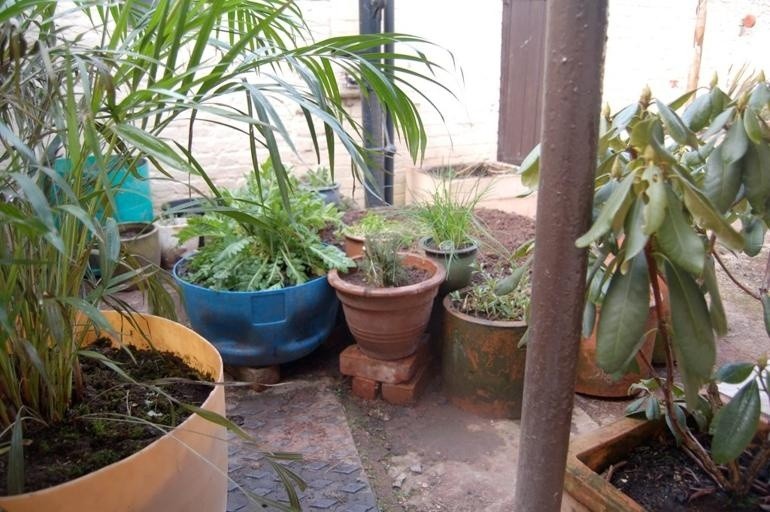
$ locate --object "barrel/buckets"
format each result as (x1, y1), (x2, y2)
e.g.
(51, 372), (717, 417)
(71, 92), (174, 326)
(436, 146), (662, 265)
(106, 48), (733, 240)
(44, 153), (152, 242)
